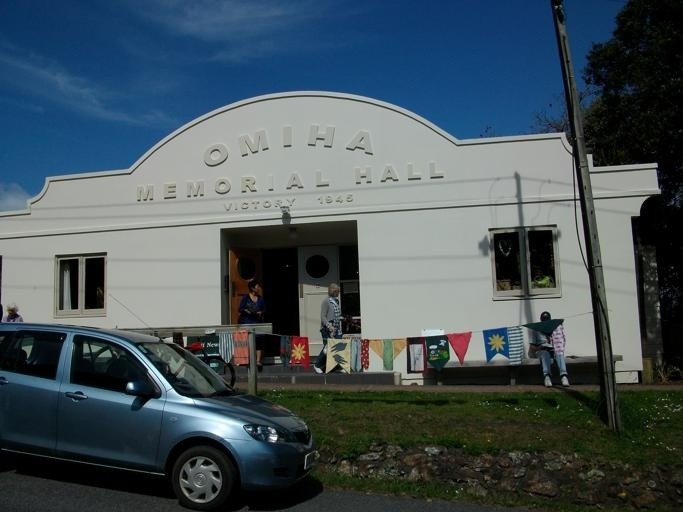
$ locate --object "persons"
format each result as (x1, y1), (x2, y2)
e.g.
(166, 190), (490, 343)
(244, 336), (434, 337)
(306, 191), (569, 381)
(313, 282), (342, 374)
(529, 311), (570, 388)
(0, 302), (24, 323)
(237, 278), (269, 366)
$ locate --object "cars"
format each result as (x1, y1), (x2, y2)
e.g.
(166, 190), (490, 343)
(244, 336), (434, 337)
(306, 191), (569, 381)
(0, 322), (320, 510)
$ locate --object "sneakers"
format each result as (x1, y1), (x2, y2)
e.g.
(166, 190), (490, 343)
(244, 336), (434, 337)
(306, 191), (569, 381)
(561, 376), (570, 387)
(313, 364), (323, 374)
(544, 376), (552, 388)
(334, 368), (348, 373)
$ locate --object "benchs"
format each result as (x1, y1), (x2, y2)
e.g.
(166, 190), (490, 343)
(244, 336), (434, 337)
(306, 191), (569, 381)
(426, 354), (623, 387)
